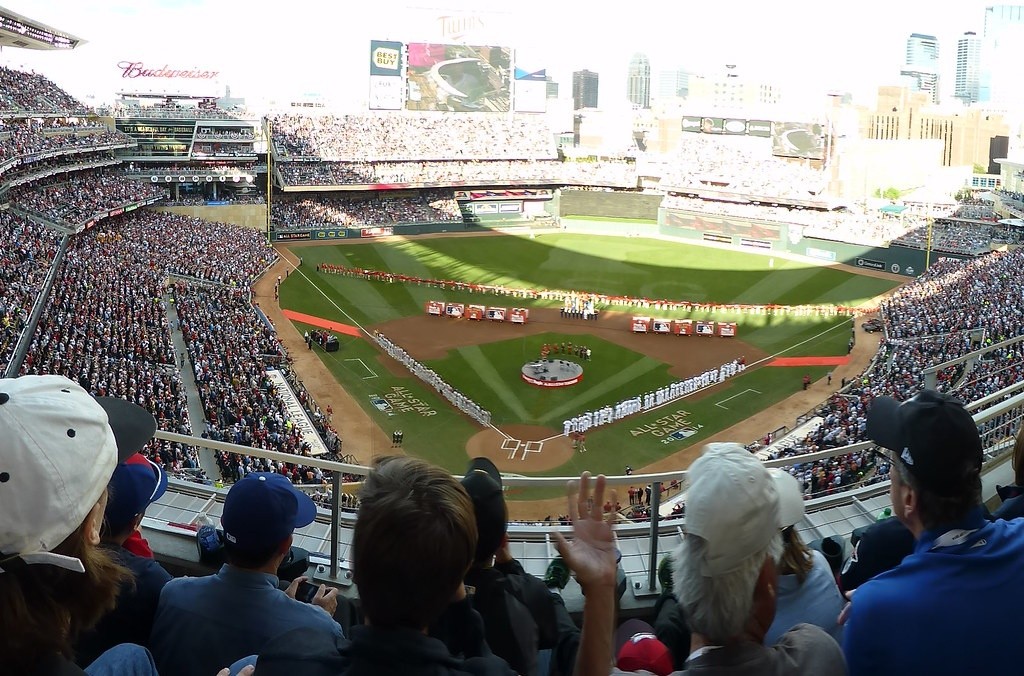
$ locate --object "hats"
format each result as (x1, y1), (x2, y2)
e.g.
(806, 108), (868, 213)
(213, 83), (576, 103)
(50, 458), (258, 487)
(109, 452), (168, 505)
(223, 472), (318, 543)
(615, 618), (672, 675)
(682, 441), (804, 576)
(0, 374), (157, 574)
(461, 457), (505, 557)
(867, 390), (984, 492)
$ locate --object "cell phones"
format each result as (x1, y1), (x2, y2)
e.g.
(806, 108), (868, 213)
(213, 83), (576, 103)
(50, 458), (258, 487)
(295, 581), (331, 604)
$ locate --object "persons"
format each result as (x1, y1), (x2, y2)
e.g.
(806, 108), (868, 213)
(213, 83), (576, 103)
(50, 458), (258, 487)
(604, 500), (611, 513)
(0, 67), (558, 512)
(625, 465), (633, 476)
(73, 454), (174, 667)
(553, 443), (847, 676)
(460, 457), (558, 676)
(628, 487), (634, 505)
(544, 541), (691, 676)
(645, 484), (651, 503)
(836, 390), (1024, 676)
(638, 488), (643, 504)
(991, 426), (1024, 523)
(631, 188), (1024, 528)
(0, 374), (254, 676)
(660, 482), (666, 503)
(761, 526), (848, 662)
(255, 455), (519, 676)
(578, 432), (587, 453)
(836, 515), (917, 602)
(149, 472), (342, 676)
(615, 502), (621, 511)
